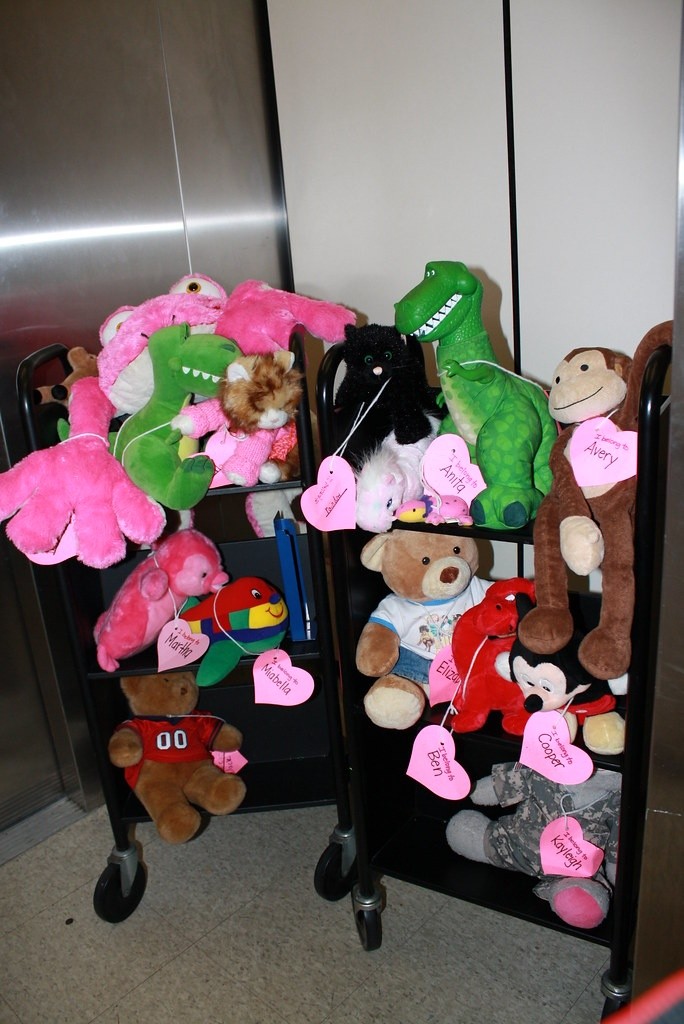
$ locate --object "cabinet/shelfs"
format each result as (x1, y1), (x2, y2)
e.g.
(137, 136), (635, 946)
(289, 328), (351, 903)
(0, 329), (674, 1024)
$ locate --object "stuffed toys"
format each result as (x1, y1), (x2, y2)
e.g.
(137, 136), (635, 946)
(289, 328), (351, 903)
(313, 260), (674, 679)
(90, 487), (309, 686)
(0, 274), (356, 570)
(107, 671), (248, 844)
(347, 529), (622, 931)
